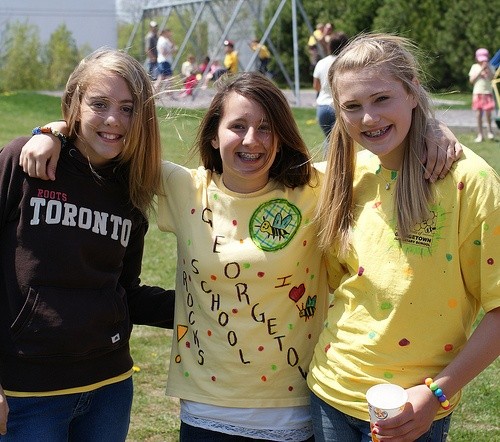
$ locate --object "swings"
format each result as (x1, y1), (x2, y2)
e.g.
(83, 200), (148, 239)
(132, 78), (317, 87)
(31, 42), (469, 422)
(194, 1), (272, 76)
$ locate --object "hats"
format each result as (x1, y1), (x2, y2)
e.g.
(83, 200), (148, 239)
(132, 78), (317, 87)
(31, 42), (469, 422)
(475, 48), (489, 62)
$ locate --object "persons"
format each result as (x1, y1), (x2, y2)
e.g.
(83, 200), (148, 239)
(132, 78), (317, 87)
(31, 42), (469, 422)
(144, 20), (158, 81)
(251, 37), (272, 75)
(169, 54), (224, 100)
(294, 29), (500, 442)
(308, 23), (335, 68)
(210, 39), (238, 81)
(0, 43), (175, 442)
(19, 28), (463, 442)
(468, 48), (496, 144)
(313, 32), (348, 161)
(153, 26), (178, 100)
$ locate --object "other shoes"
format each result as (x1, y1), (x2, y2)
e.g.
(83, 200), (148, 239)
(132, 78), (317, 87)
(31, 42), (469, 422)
(475, 136), (483, 142)
(487, 132), (494, 139)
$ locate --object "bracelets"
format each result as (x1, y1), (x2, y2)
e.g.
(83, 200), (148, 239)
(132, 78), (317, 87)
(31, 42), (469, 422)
(32, 125), (66, 147)
(425, 378), (450, 410)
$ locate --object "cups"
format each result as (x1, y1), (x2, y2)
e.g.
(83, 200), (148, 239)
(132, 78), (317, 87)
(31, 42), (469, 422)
(366, 384), (408, 442)
